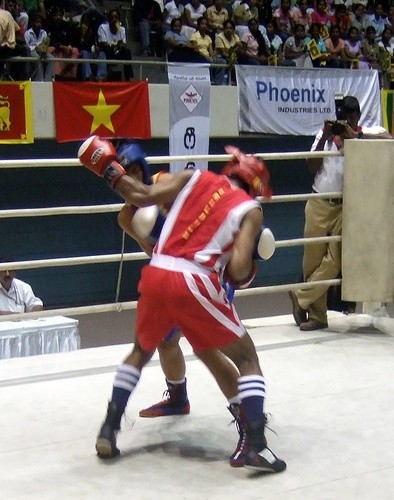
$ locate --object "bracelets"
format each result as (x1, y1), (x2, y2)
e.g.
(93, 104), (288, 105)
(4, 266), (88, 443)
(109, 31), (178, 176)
(356, 132), (363, 140)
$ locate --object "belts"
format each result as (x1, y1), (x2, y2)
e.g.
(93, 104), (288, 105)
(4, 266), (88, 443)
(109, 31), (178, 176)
(311, 188), (343, 205)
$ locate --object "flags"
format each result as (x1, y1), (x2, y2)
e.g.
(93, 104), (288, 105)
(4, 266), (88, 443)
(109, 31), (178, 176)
(51, 80), (152, 144)
(0, 81), (34, 144)
(235, 64), (382, 135)
(166, 64), (212, 175)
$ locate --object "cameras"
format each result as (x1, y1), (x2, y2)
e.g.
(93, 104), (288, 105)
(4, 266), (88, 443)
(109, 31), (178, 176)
(326, 120), (345, 135)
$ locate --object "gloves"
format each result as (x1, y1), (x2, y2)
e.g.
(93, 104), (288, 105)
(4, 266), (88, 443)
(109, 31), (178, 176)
(222, 262), (258, 289)
(131, 206), (166, 245)
(251, 227), (276, 261)
(78, 136), (127, 190)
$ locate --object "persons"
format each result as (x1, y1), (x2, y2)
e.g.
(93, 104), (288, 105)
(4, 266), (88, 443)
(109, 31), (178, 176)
(77, 136), (287, 473)
(0, 271), (42, 316)
(289, 96), (394, 331)
(0, 0), (394, 85)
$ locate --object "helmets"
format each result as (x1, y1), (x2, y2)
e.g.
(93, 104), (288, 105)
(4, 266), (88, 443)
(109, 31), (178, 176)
(219, 145), (273, 199)
(117, 144), (153, 186)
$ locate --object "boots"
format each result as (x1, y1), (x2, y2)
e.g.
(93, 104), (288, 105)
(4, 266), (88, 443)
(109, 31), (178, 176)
(139, 377), (190, 417)
(243, 413), (288, 472)
(96, 402), (125, 459)
(227, 403), (248, 467)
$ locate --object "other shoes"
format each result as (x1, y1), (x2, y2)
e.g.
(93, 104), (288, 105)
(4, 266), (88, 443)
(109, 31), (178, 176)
(300, 317), (328, 331)
(289, 290), (306, 326)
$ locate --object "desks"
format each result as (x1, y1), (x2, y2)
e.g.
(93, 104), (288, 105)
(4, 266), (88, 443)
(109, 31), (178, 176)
(0, 316), (81, 358)
